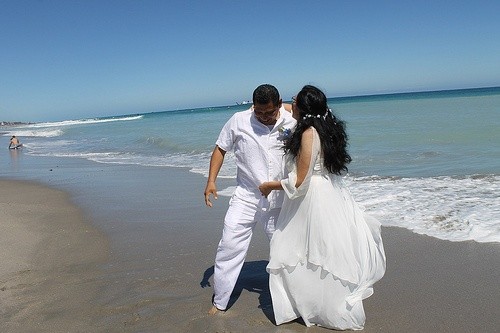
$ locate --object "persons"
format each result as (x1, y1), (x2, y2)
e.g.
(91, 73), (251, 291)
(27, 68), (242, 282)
(10, 136), (23, 149)
(204, 83), (302, 317)
(251, 85), (386, 331)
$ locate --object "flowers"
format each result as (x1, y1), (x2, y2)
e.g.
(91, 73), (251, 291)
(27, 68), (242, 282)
(278, 126), (290, 137)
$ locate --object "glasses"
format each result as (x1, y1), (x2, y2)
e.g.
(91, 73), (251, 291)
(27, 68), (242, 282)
(292, 96), (297, 101)
(254, 109), (276, 115)
